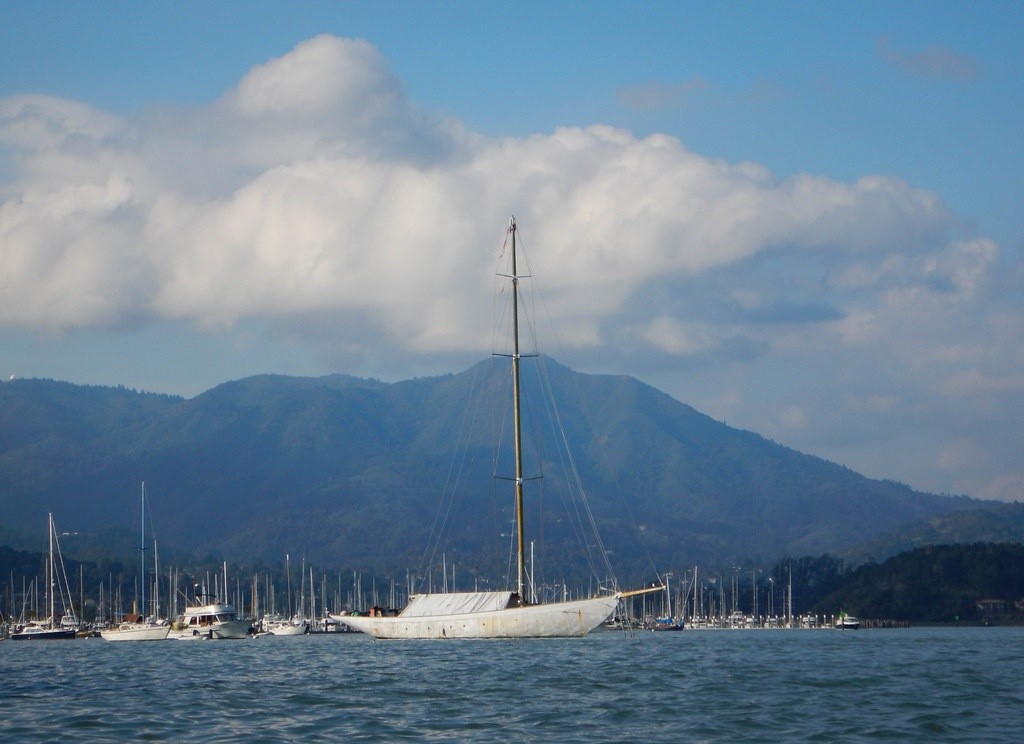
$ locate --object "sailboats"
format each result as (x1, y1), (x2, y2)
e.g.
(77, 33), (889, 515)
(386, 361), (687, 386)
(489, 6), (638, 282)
(0, 478), (861, 639)
(329, 211), (667, 639)
